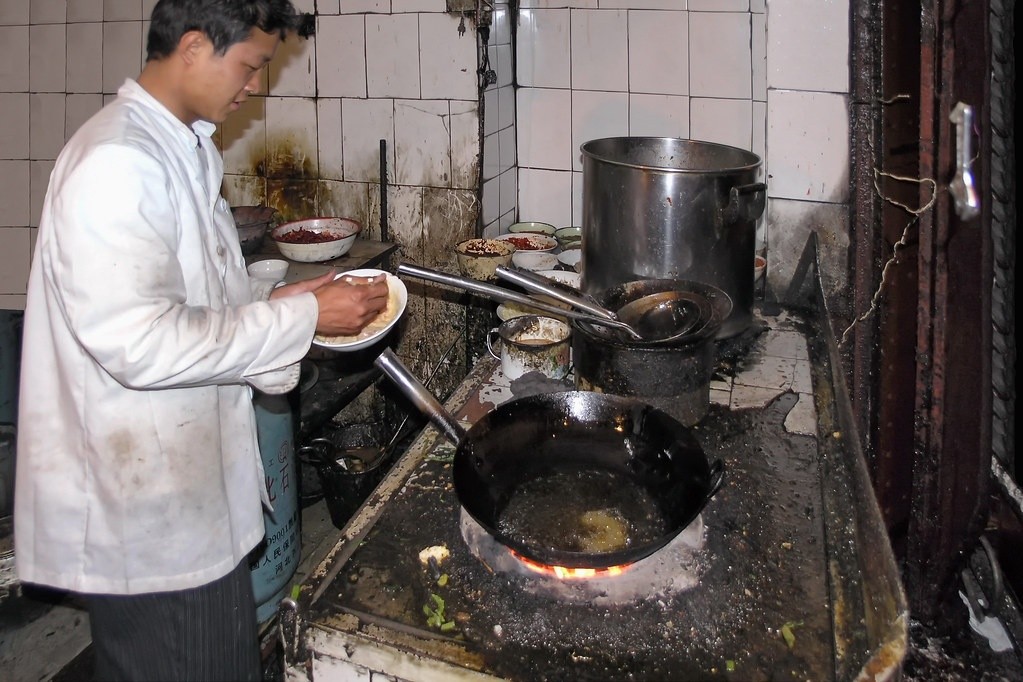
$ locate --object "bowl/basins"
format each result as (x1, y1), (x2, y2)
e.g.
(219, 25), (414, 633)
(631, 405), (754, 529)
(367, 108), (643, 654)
(557, 249), (581, 266)
(525, 270), (581, 297)
(271, 216), (360, 263)
(454, 238), (515, 282)
(495, 232), (558, 253)
(561, 241), (581, 252)
(247, 259), (289, 282)
(512, 252), (558, 271)
(553, 227), (581, 245)
(508, 222), (557, 237)
(496, 294), (571, 325)
(754, 255), (766, 283)
(313, 269), (408, 352)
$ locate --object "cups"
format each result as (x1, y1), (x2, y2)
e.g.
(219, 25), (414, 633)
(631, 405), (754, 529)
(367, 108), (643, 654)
(487, 316), (570, 381)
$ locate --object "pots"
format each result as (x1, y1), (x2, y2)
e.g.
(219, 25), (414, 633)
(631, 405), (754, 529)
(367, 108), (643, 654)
(373, 347), (726, 571)
(579, 137), (766, 339)
(516, 268), (733, 345)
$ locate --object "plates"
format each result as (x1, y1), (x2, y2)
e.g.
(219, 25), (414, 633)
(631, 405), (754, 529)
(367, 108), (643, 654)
(300, 359), (319, 393)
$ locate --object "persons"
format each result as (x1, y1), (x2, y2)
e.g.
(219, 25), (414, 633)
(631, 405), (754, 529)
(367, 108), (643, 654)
(10, 0), (390, 682)
